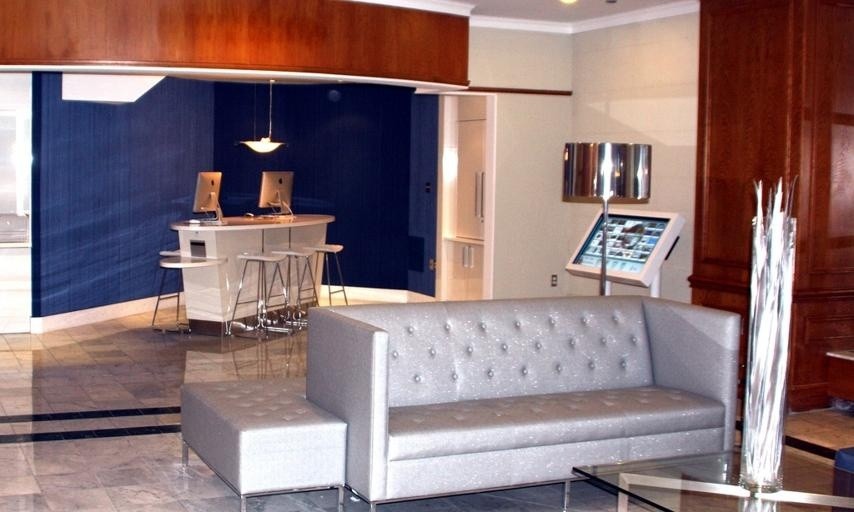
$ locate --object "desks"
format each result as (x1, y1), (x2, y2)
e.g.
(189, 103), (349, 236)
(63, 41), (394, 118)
(170, 214), (334, 336)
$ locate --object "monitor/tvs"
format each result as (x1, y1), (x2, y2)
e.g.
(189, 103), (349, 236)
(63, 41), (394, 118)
(566, 205), (686, 288)
(259, 169), (298, 215)
(191, 169), (226, 223)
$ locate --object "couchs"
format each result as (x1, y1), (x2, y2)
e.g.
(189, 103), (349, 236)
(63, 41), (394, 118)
(307, 296), (741, 512)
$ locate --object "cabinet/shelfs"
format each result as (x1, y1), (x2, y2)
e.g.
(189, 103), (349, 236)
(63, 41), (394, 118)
(446, 96), (487, 301)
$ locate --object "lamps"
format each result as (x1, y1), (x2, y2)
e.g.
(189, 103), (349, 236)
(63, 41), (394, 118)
(240, 79), (285, 154)
(562, 143), (653, 294)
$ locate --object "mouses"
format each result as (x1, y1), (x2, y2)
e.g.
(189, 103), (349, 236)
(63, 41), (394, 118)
(245, 212), (254, 217)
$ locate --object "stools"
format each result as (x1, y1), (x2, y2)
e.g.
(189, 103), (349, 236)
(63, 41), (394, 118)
(181, 380), (347, 511)
(152, 244), (349, 341)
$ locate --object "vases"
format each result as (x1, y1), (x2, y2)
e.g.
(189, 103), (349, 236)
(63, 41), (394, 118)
(738, 216), (797, 494)
